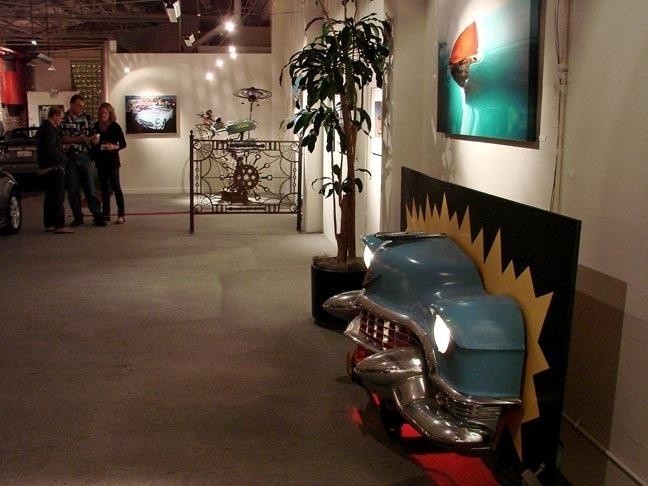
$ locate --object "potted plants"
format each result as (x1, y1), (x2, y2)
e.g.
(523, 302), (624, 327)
(279, 1), (392, 333)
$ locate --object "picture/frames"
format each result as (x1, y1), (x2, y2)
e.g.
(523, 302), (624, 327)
(38, 105), (64, 126)
(436, 0), (540, 145)
(121, 94), (180, 138)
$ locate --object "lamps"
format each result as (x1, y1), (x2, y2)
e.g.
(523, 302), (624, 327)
(181, 24), (197, 47)
(163, 0), (181, 22)
(26, 0), (60, 72)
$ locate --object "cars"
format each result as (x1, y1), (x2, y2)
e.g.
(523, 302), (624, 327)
(0, 119), (38, 233)
(137, 105), (174, 130)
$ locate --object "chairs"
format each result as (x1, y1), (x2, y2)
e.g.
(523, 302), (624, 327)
(9, 133), (37, 146)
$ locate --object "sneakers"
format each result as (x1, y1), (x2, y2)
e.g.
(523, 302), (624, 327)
(46, 214), (129, 237)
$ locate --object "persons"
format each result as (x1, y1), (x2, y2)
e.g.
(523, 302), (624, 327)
(84, 103), (126, 225)
(57, 93), (109, 227)
(32, 103), (91, 235)
